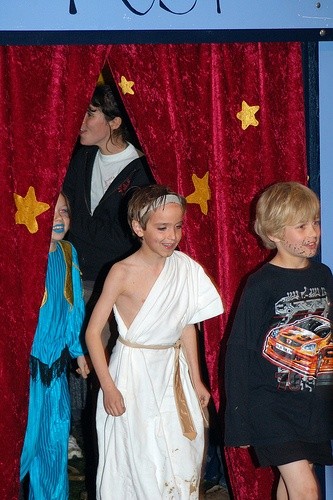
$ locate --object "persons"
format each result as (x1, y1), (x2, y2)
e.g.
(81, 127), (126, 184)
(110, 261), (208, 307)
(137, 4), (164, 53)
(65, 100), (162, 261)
(85, 182), (224, 500)
(225, 181), (333, 500)
(63, 82), (158, 360)
(19, 188), (90, 500)
